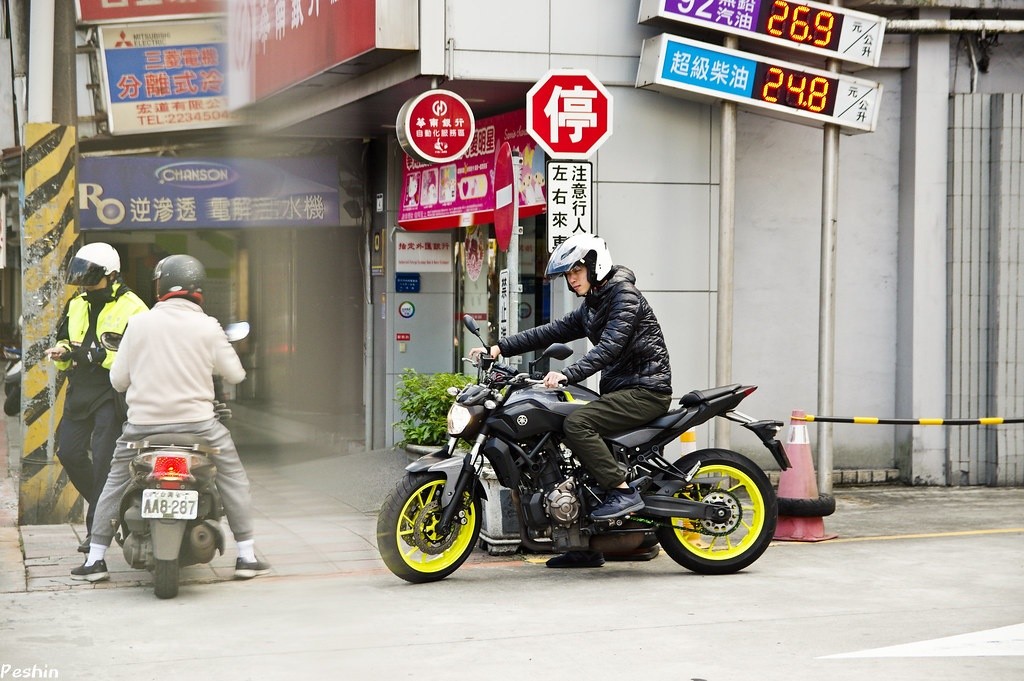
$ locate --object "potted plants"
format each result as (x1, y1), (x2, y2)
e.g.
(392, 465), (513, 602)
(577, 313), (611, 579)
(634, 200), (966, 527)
(390, 367), (535, 556)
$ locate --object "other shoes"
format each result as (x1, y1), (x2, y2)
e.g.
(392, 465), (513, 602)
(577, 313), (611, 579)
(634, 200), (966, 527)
(77, 535), (91, 552)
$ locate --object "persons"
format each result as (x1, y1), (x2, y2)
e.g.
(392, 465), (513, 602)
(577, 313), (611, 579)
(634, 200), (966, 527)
(44, 242), (149, 553)
(470, 233), (673, 568)
(69, 257), (272, 581)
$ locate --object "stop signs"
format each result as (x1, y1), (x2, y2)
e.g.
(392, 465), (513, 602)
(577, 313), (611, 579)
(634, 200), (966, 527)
(525, 69), (612, 161)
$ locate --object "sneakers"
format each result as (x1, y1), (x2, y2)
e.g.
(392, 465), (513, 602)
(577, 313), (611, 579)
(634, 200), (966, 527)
(235, 554), (270, 577)
(591, 484), (644, 520)
(71, 559), (109, 581)
(546, 551), (605, 568)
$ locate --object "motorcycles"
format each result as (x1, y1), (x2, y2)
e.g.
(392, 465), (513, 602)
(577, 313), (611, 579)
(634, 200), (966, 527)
(376, 314), (793, 584)
(100, 320), (250, 598)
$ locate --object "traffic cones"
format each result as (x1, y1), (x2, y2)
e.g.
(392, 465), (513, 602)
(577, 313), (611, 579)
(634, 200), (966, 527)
(773, 410), (838, 543)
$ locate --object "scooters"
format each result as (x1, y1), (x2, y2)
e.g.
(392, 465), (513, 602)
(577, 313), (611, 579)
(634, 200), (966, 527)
(2, 346), (23, 416)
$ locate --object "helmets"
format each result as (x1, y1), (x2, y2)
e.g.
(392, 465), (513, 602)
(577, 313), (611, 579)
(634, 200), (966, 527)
(544, 234), (613, 292)
(65, 241), (121, 285)
(153, 252), (207, 306)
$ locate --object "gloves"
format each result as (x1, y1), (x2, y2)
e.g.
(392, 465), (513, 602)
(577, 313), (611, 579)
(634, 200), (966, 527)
(66, 343), (94, 368)
(212, 400), (232, 421)
